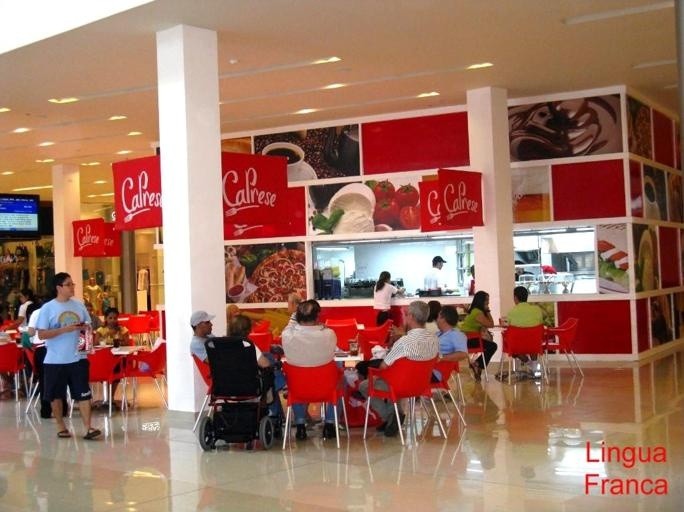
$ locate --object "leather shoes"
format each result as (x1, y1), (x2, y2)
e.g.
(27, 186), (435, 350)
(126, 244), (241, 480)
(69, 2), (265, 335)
(295, 427), (306, 440)
(322, 425), (336, 438)
(385, 413), (404, 437)
(375, 421), (387, 431)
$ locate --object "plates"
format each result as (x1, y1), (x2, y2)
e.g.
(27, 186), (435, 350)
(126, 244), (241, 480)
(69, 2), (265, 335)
(287, 162), (323, 180)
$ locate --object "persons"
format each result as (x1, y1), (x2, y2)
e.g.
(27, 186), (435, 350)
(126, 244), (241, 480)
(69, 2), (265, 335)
(0, 272), (129, 439)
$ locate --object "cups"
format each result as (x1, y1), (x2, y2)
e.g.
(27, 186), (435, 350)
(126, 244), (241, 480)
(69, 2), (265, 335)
(113, 338), (120, 348)
(260, 141), (304, 181)
(349, 343), (358, 356)
(643, 177), (662, 220)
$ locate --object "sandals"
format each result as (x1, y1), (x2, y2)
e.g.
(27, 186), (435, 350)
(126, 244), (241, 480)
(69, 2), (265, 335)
(469, 363), (482, 381)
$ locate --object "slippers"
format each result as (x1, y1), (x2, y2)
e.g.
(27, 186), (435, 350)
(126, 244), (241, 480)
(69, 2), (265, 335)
(83, 428), (102, 440)
(57, 429), (71, 437)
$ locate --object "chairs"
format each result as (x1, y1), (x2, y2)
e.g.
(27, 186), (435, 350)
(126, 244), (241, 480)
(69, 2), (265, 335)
(1, 308), (586, 451)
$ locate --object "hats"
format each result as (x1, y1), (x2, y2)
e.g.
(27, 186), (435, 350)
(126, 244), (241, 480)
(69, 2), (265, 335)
(432, 256), (446, 263)
(190, 311), (216, 327)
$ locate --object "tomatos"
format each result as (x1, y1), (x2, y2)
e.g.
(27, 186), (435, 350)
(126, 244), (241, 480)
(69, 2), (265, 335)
(366, 181), (420, 229)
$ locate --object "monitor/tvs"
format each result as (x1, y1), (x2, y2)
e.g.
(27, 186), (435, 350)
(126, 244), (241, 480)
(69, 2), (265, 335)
(0, 193), (40, 242)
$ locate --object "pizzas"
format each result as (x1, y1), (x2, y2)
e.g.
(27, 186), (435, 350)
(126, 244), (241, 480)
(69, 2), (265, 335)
(247, 250), (307, 303)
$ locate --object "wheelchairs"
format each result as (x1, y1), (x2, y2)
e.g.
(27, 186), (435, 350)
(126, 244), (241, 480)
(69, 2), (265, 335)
(198, 335), (282, 451)
